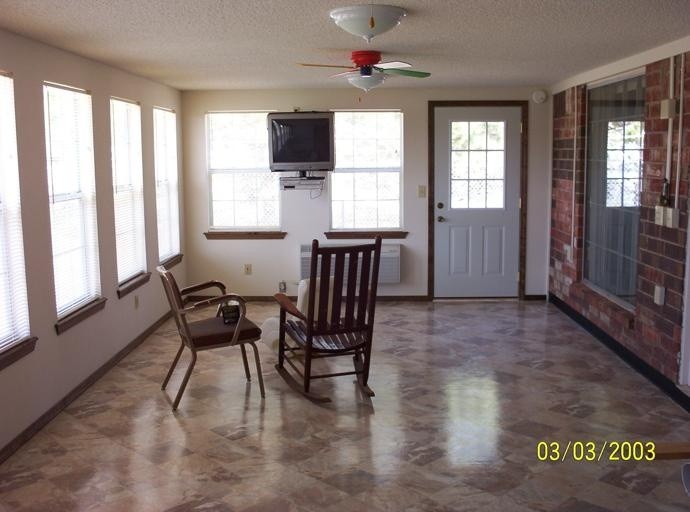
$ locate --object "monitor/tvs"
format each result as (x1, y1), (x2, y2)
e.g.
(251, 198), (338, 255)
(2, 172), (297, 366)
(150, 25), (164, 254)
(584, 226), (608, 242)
(267, 111), (335, 181)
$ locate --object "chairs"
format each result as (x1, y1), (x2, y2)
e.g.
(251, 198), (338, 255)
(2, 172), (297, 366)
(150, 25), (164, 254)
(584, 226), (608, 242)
(274, 234), (383, 402)
(155, 265), (266, 411)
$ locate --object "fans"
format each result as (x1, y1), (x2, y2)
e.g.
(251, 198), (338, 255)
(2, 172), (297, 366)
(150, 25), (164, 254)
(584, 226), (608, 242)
(295, 50), (432, 92)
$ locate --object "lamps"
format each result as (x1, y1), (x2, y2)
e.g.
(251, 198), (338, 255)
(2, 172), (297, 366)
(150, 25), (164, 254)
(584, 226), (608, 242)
(345, 74), (386, 93)
(330, 3), (408, 44)
